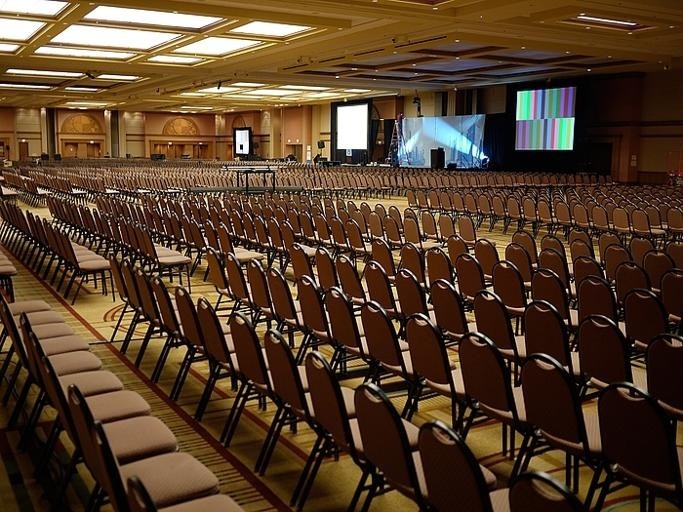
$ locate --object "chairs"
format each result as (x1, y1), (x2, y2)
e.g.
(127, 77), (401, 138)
(0, 159), (682, 512)
